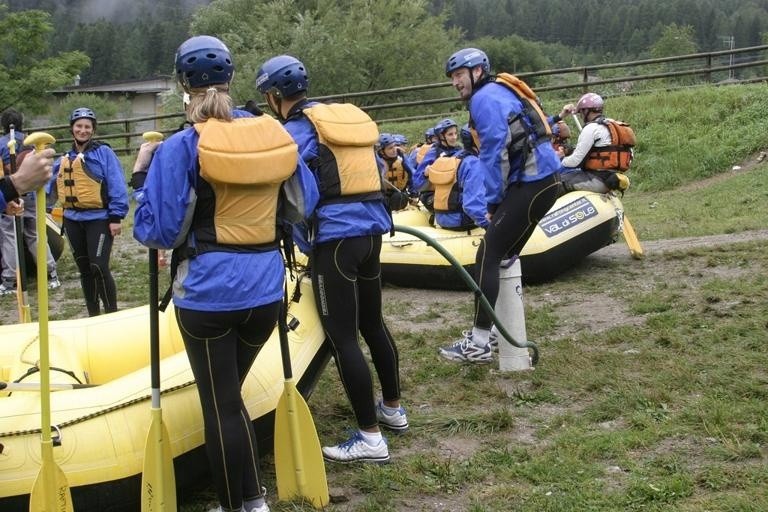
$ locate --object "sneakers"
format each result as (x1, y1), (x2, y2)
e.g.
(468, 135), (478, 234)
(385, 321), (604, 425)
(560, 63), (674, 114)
(241, 501), (272, 512)
(437, 331), (494, 363)
(488, 329), (500, 353)
(374, 399), (410, 432)
(48, 278), (61, 291)
(208, 504), (226, 512)
(321, 429), (392, 463)
(0, 283), (18, 296)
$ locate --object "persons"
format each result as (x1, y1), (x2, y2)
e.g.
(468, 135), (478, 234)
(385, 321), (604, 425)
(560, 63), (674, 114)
(548, 90), (633, 197)
(0, 147), (56, 212)
(0, 107), (61, 296)
(52, 106), (129, 317)
(377, 118), (490, 237)
(254, 57), (408, 462)
(435, 48), (562, 364)
(131, 35), (323, 512)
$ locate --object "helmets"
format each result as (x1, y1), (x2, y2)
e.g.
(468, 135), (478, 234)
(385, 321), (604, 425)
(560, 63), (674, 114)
(575, 92), (605, 115)
(255, 54), (309, 101)
(445, 46), (491, 77)
(175, 33), (236, 89)
(375, 117), (478, 150)
(70, 106), (97, 125)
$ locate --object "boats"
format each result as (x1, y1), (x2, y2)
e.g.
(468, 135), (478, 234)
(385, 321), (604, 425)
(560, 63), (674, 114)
(0, 242), (334, 512)
(377, 192), (625, 291)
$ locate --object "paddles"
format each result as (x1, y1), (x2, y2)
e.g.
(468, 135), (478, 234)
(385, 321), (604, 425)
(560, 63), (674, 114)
(570, 109), (644, 260)
(24, 132), (75, 512)
(274, 301), (330, 509)
(140, 131), (178, 512)
(9, 124), (31, 323)
